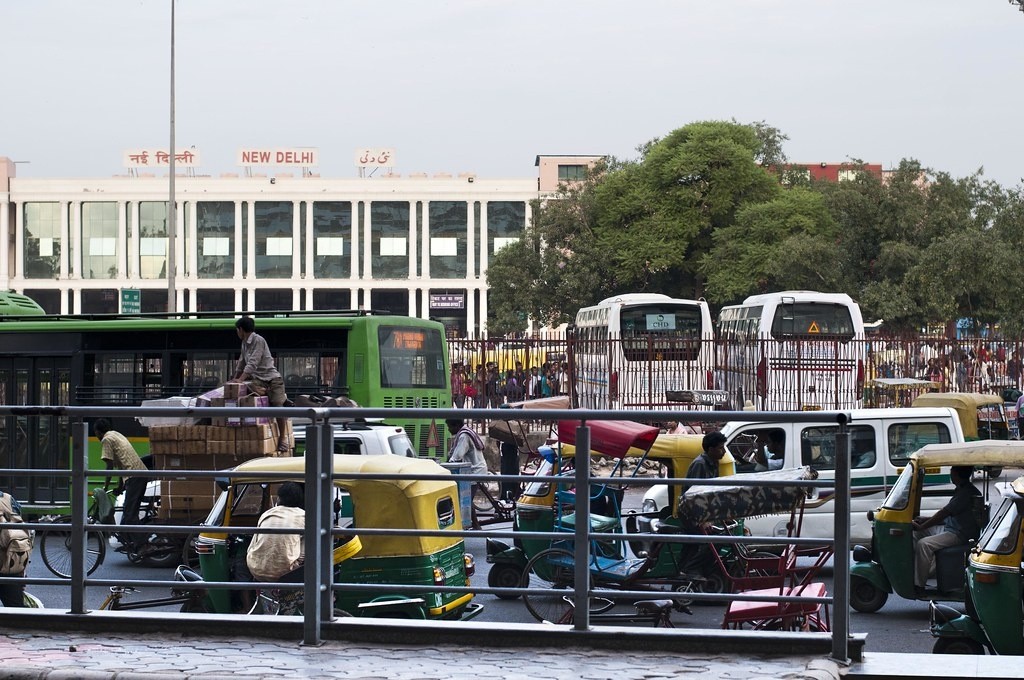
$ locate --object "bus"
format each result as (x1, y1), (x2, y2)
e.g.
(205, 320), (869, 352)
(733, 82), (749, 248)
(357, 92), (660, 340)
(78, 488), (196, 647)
(571, 294), (716, 412)
(716, 290), (866, 410)
(0, 294), (454, 514)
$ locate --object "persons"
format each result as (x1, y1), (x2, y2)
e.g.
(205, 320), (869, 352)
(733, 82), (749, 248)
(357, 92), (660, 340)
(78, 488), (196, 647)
(671, 430), (733, 615)
(753, 429), (784, 471)
(852, 430), (875, 467)
(446, 419), (488, 530)
(452, 359), (569, 408)
(665, 420), (683, 434)
(864, 338), (1024, 408)
(499, 404), (523, 501)
(228, 317), (289, 452)
(1015, 395), (1024, 439)
(912, 466), (984, 591)
(246, 481), (305, 615)
(0, 490), (24, 608)
(93, 418), (149, 552)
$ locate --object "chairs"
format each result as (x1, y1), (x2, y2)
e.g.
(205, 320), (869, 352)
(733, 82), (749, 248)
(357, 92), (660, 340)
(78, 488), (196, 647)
(934, 496), (982, 592)
(283, 373), (301, 398)
(198, 375), (219, 394)
(180, 375), (202, 396)
(294, 375), (317, 399)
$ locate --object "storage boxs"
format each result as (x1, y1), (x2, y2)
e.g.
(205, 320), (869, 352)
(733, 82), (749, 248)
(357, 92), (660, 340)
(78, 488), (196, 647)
(133, 378), (296, 524)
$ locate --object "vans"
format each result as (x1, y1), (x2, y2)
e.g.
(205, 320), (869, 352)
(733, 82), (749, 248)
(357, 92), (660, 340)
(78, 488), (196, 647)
(109, 417), (417, 548)
(640, 407), (965, 548)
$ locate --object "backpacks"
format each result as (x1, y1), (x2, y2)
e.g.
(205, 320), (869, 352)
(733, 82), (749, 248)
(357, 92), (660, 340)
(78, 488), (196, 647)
(0, 493), (36, 575)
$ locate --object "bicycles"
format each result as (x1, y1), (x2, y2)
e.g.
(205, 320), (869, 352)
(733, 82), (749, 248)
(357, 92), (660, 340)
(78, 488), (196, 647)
(246, 568), (351, 618)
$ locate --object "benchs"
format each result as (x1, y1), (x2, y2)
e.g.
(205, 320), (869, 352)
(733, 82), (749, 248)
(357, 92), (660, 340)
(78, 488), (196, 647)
(724, 542), (827, 622)
(553, 490), (627, 570)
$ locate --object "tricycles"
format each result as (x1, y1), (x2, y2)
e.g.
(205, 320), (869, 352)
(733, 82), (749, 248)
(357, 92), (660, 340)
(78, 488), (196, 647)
(40, 485), (209, 579)
(474, 395), (569, 525)
(520, 417), (799, 624)
(541, 465), (833, 633)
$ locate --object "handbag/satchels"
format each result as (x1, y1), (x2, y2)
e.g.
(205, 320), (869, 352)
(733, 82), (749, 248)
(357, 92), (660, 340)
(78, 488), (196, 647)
(463, 386), (477, 399)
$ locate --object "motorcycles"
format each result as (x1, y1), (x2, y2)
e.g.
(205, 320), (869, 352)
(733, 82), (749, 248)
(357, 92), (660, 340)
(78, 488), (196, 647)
(846, 439), (1024, 614)
(928, 475), (1024, 656)
(486, 434), (737, 599)
(906, 392), (1009, 477)
(180, 456), (484, 621)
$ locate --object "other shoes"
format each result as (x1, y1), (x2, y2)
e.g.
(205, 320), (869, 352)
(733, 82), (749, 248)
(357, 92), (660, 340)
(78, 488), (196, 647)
(277, 442), (289, 452)
(914, 583), (925, 597)
(678, 571), (708, 581)
(131, 542), (157, 561)
(114, 543), (134, 552)
